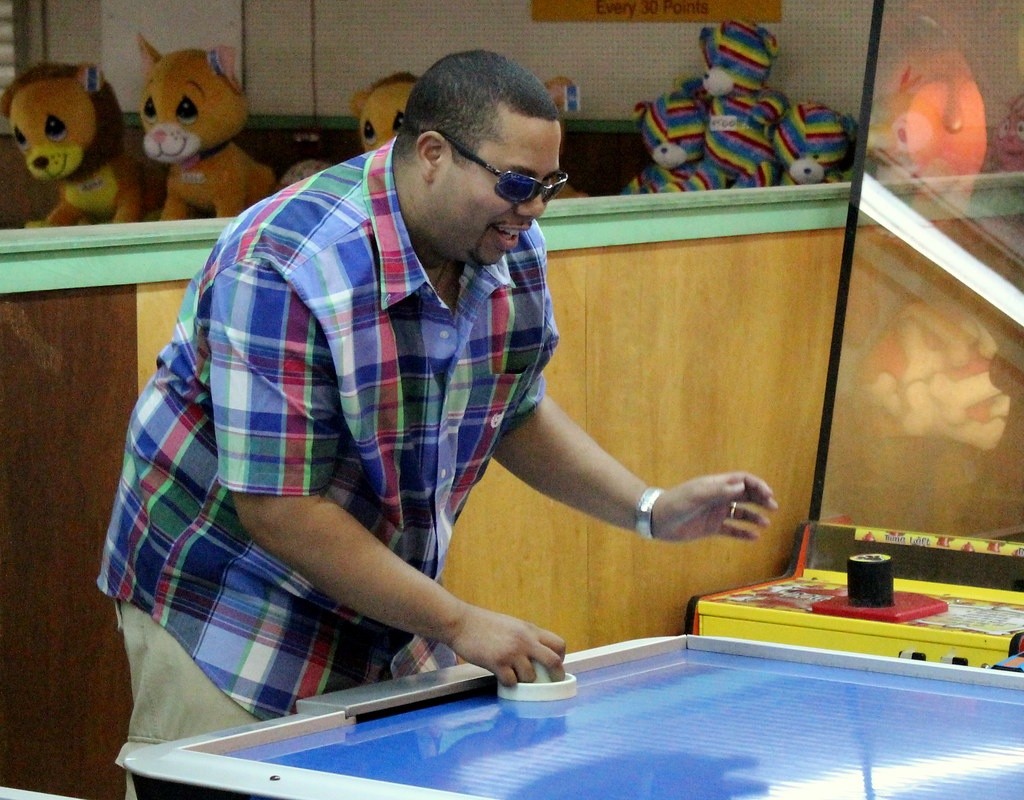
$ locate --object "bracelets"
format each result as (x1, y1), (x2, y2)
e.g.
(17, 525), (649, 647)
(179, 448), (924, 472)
(634, 485), (667, 541)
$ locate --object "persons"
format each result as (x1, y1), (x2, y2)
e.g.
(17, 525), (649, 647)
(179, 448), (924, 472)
(97, 52), (776, 800)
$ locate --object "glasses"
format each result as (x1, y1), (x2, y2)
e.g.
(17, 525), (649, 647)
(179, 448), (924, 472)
(416, 129), (570, 202)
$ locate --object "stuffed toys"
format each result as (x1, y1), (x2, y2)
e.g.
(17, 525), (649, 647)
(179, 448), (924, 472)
(864, 65), (961, 188)
(765, 101), (860, 187)
(237, 119), (359, 190)
(673, 20), (787, 186)
(4, 58), (167, 224)
(131, 35), (275, 223)
(620, 76), (721, 194)
(346, 67), (415, 154)
(992, 87), (1024, 172)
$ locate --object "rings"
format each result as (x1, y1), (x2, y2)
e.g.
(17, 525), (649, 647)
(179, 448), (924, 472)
(730, 503), (739, 518)
